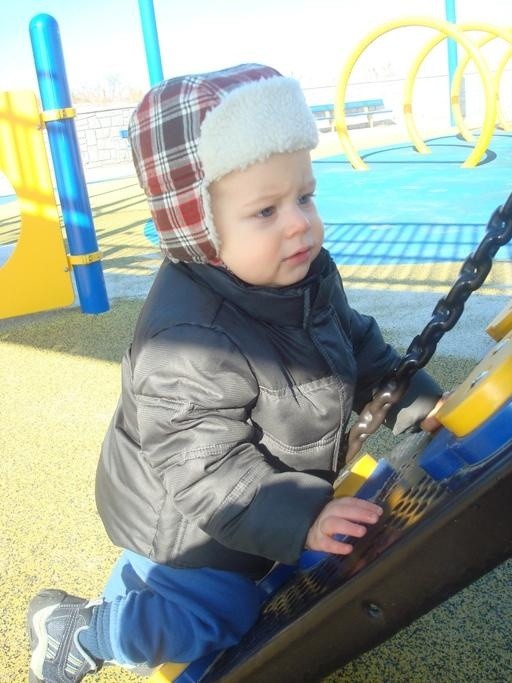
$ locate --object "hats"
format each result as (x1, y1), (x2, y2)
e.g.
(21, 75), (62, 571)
(127, 64), (318, 264)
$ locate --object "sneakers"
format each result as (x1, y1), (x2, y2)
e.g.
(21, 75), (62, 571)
(27, 590), (104, 683)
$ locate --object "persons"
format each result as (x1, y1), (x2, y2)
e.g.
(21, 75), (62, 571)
(27, 65), (455, 683)
